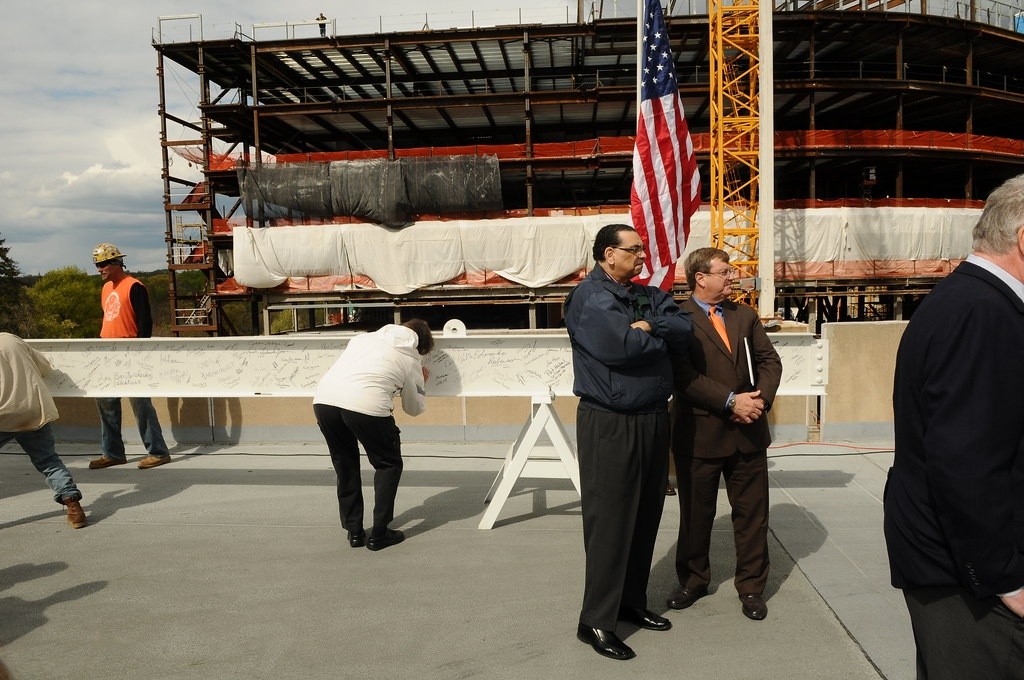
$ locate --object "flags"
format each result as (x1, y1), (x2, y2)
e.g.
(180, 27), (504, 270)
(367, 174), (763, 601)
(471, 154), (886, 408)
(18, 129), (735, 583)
(630, 0), (701, 294)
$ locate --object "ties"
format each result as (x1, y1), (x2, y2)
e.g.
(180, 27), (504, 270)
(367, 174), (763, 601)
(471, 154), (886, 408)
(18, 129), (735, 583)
(708, 306), (732, 353)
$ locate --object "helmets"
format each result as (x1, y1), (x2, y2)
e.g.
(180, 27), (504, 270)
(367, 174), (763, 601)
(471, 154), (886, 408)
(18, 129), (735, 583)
(91, 243), (126, 264)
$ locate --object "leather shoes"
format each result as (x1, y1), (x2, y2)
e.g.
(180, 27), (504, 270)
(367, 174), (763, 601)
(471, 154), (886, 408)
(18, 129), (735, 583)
(577, 622), (635, 660)
(346, 528), (367, 547)
(367, 530), (405, 551)
(666, 584), (709, 609)
(617, 605), (672, 631)
(739, 594), (767, 620)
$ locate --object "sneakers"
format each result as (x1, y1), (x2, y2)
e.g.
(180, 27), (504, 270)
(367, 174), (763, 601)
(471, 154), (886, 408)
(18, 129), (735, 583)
(63, 501), (87, 529)
(137, 454), (171, 468)
(89, 454), (128, 470)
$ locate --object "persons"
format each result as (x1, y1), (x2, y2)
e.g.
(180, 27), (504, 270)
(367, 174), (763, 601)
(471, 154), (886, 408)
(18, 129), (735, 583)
(0, 331), (87, 529)
(665, 249), (783, 621)
(561, 225), (693, 659)
(88, 244), (171, 470)
(313, 319), (434, 551)
(883, 173), (1024, 680)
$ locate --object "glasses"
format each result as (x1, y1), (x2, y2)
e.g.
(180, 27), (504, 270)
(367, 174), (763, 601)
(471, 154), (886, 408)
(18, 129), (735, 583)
(612, 245), (646, 255)
(703, 268), (734, 279)
(95, 260), (117, 267)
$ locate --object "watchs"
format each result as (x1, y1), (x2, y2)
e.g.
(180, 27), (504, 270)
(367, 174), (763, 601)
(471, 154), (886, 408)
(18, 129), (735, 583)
(727, 394), (736, 409)
